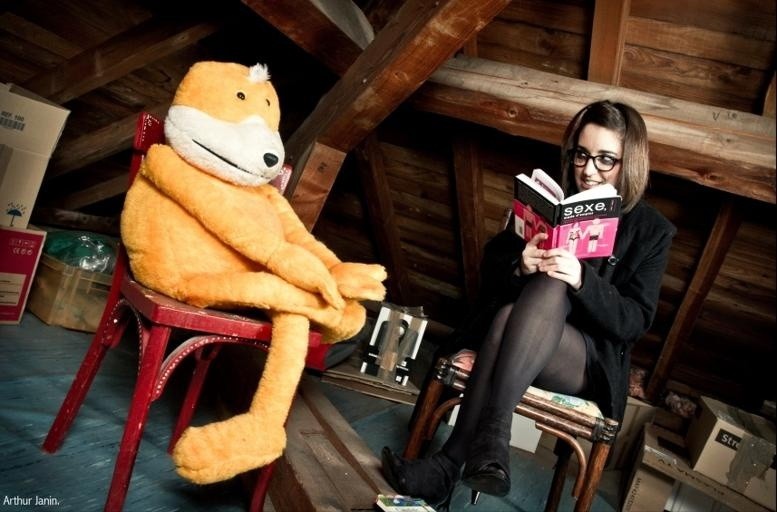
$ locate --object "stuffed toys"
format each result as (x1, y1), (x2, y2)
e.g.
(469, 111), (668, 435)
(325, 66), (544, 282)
(120, 60), (387, 484)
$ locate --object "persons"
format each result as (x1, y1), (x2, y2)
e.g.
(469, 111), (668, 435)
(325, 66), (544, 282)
(375, 99), (676, 512)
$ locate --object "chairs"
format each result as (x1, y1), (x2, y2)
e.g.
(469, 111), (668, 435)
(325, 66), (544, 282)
(405, 348), (619, 511)
(43, 113), (321, 512)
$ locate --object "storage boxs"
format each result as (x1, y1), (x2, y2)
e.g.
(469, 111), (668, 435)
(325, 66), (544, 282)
(0, 227), (48, 325)
(621, 423), (770, 512)
(30, 254), (114, 334)
(538, 396), (652, 472)
(1, 83), (70, 230)
(691, 394), (777, 512)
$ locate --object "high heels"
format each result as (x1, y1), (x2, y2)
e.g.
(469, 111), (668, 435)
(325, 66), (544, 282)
(379, 443), (458, 511)
(458, 443), (511, 506)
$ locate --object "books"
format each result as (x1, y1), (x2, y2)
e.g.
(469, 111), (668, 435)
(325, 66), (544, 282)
(515, 169), (622, 259)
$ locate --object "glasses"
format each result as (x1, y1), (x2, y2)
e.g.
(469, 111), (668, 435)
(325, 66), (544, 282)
(572, 146), (624, 173)
